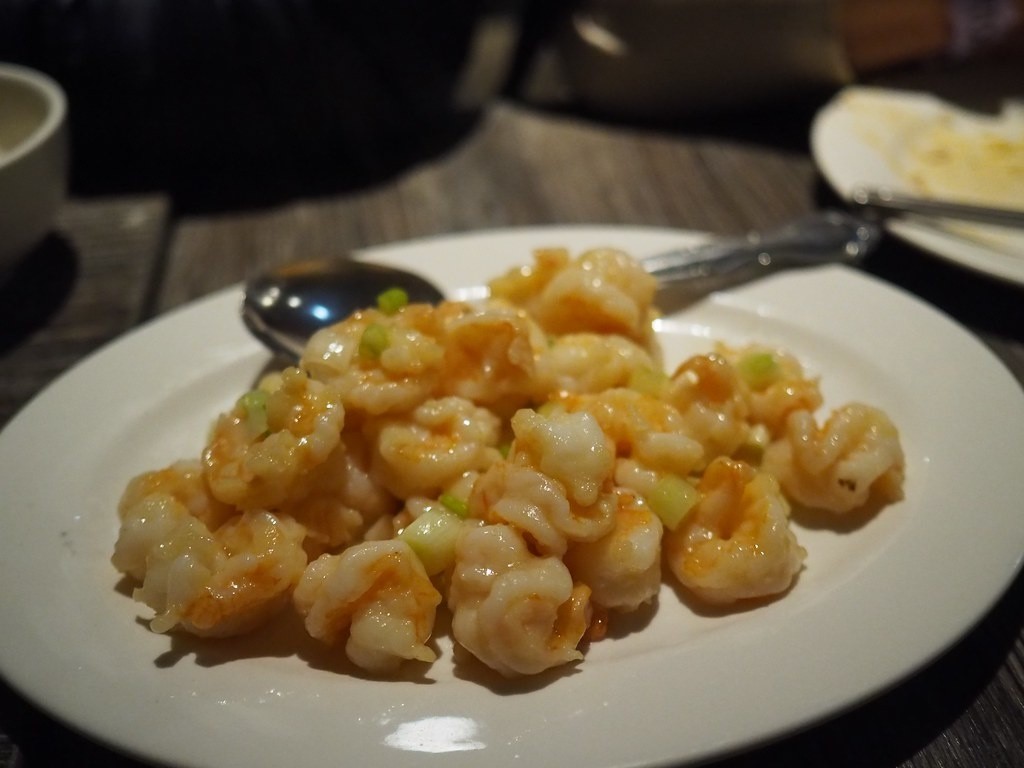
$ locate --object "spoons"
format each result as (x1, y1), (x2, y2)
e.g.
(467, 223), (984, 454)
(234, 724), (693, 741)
(241, 212), (882, 368)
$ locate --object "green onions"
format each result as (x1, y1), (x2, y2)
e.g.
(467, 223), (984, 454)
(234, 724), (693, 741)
(236, 288), (784, 579)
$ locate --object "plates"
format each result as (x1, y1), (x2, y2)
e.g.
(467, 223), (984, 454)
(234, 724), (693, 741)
(0, 222), (1024, 768)
(811, 87), (1024, 287)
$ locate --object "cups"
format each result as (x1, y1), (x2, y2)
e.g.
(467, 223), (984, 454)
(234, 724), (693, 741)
(0, 60), (68, 270)
